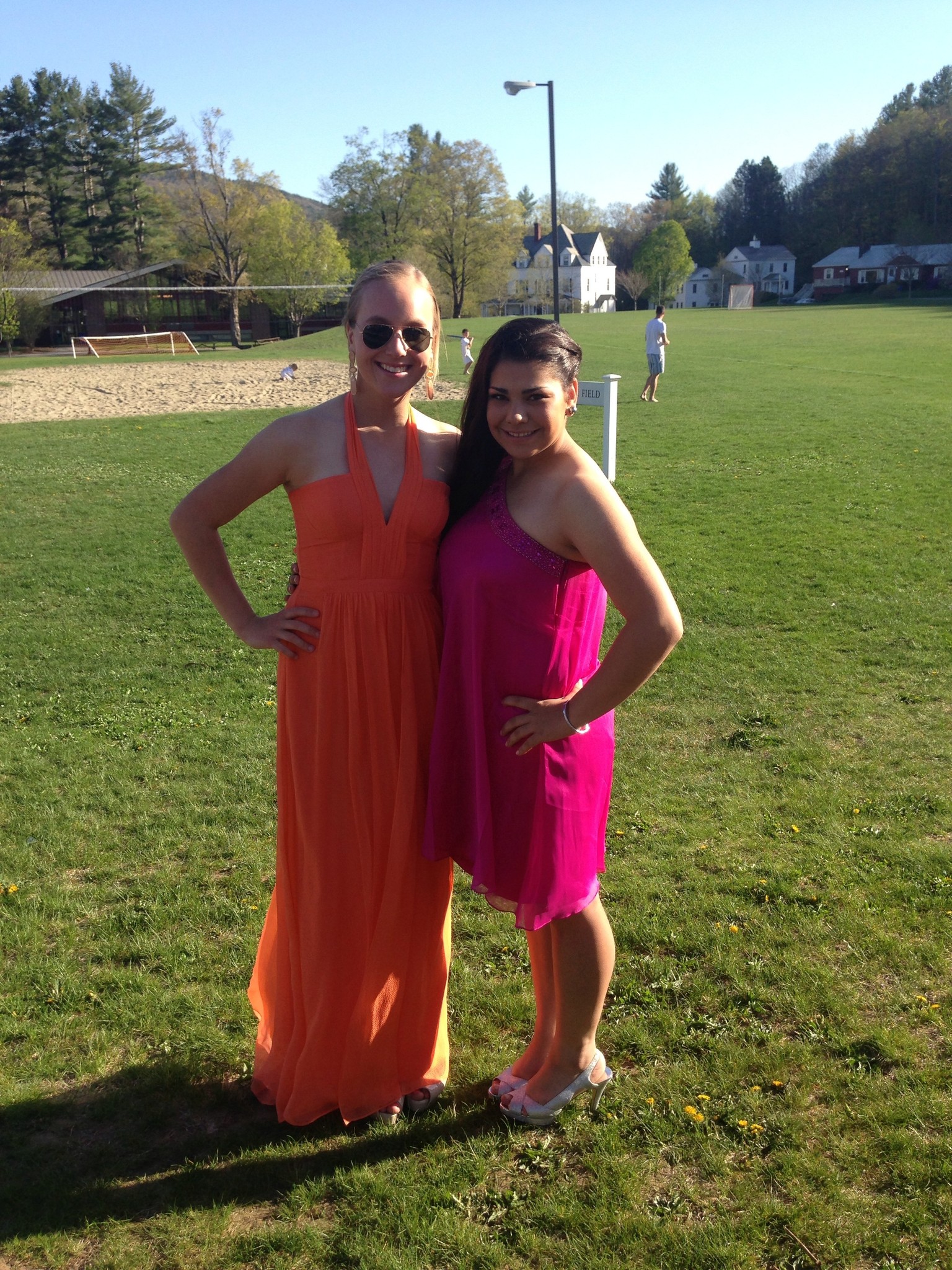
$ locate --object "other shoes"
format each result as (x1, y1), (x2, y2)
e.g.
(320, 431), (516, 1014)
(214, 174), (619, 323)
(464, 371), (470, 374)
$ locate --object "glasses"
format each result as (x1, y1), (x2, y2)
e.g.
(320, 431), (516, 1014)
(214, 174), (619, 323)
(353, 320), (435, 352)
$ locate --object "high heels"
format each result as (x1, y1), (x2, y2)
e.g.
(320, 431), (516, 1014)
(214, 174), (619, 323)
(374, 1077), (449, 1124)
(488, 1042), (613, 1125)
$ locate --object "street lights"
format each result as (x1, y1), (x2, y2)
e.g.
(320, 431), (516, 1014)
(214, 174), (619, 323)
(845, 267), (848, 303)
(504, 79), (560, 325)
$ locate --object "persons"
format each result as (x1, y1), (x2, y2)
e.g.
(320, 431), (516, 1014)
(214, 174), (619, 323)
(639, 304), (671, 403)
(458, 328), (475, 376)
(280, 363), (299, 381)
(168, 257), (481, 1130)
(425, 318), (687, 1126)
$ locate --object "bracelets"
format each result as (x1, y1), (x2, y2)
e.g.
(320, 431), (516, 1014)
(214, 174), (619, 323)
(560, 698), (593, 735)
